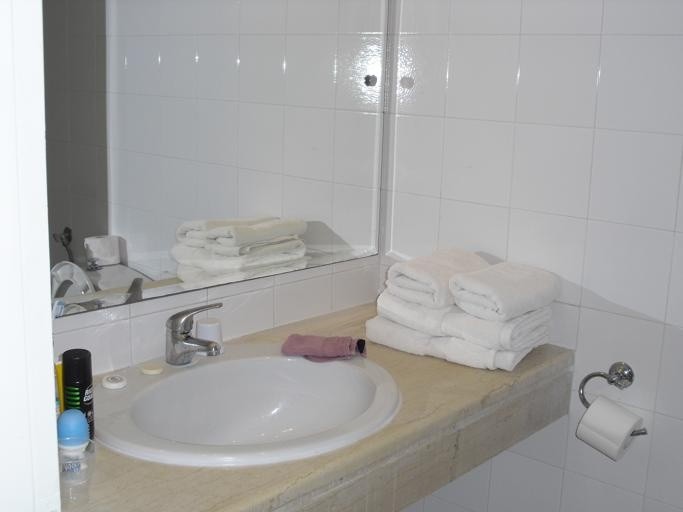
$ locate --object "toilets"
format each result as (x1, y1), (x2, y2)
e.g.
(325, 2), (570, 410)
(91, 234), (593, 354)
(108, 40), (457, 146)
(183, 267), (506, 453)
(51, 263), (152, 295)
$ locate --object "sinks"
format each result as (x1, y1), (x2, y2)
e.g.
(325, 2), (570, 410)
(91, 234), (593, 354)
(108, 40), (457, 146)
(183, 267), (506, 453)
(90, 343), (401, 469)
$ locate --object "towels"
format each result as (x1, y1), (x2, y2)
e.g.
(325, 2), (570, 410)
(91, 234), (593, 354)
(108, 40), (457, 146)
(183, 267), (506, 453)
(362, 249), (561, 373)
(280, 333), (369, 365)
(171, 214), (309, 282)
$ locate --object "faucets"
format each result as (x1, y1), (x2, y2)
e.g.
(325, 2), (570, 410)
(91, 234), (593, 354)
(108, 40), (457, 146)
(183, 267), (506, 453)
(163, 302), (222, 362)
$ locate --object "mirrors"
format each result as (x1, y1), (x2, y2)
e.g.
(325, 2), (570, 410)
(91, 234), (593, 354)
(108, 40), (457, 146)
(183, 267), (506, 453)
(40, 1), (388, 319)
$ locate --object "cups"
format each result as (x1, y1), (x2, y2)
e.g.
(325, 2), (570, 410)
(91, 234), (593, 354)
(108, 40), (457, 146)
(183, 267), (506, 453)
(58, 437), (95, 512)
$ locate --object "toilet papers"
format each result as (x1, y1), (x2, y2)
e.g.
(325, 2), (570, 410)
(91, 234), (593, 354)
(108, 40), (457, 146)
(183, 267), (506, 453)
(82, 235), (122, 268)
(575, 394), (642, 461)
